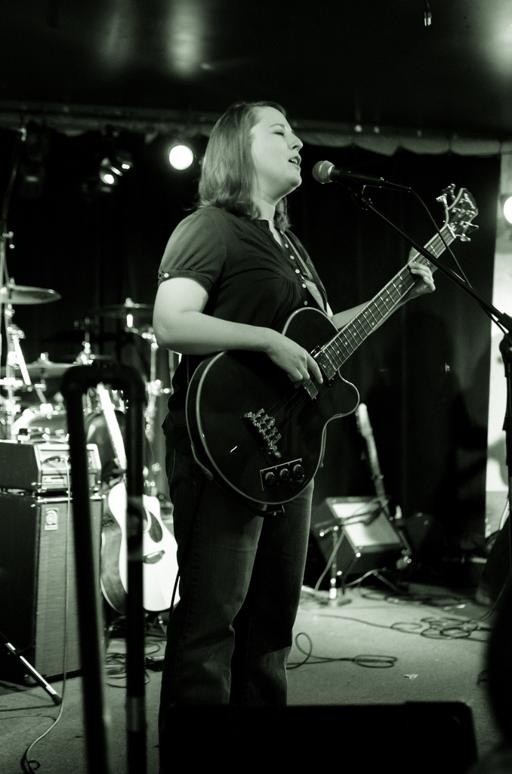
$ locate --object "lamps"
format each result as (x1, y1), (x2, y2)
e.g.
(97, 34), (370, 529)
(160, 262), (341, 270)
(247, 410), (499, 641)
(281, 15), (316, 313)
(0, 107), (199, 193)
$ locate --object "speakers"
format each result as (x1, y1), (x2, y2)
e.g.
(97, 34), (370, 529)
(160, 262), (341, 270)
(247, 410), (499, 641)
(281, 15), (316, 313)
(0, 496), (104, 684)
(310, 496), (402, 577)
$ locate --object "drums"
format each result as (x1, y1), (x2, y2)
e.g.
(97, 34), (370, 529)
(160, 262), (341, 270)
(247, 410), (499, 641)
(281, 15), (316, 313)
(27, 408), (152, 485)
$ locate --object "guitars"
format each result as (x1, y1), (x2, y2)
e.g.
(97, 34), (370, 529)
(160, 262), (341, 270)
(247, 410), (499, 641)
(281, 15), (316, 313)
(185, 183), (479, 505)
(80, 341), (180, 615)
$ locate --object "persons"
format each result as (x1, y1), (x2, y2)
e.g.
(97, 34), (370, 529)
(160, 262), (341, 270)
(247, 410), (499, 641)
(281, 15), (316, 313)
(150, 96), (436, 772)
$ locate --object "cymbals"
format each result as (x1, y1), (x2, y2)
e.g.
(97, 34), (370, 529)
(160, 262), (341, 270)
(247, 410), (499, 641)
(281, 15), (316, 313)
(98, 304), (153, 318)
(0, 285), (59, 305)
(1, 363), (80, 379)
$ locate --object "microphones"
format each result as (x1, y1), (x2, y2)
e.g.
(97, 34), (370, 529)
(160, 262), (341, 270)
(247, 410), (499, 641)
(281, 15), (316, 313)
(396, 556), (412, 570)
(312, 160), (411, 193)
(364, 499), (382, 525)
